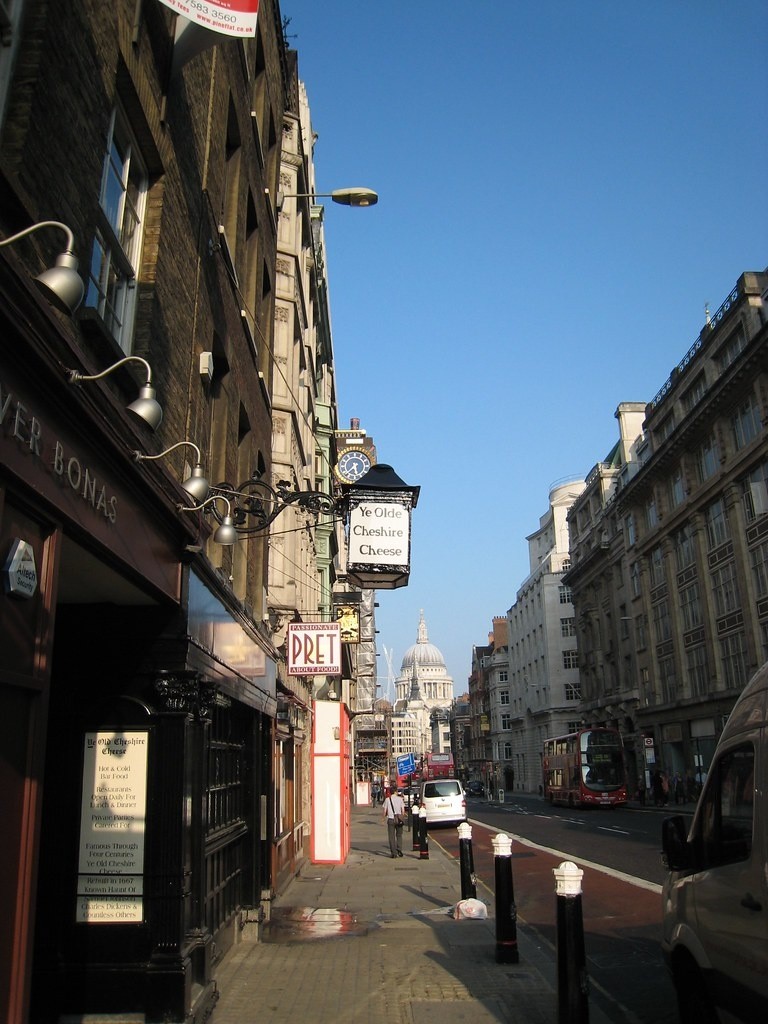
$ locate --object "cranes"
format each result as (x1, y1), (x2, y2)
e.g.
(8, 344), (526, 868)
(378, 643), (397, 702)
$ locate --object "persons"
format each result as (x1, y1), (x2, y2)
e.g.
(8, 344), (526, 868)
(382, 786), (405, 858)
(653, 766), (707, 808)
(386, 780), (396, 798)
(370, 783), (381, 808)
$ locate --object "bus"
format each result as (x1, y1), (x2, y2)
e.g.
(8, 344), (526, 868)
(542, 727), (630, 810)
(422, 752), (456, 782)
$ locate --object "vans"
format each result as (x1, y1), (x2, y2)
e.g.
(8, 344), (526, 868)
(657, 661), (768, 1024)
(418, 778), (468, 828)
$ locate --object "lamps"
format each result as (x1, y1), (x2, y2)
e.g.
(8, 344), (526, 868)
(199, 464), (421, 590)
(136, 442), (210, 504)
(620, 617), (643, 621)
(176, 496), (238, 546)
(530, 683), (550, 690)
(69, 355), (163, 433)
(275, 187), (379, 212)
(0, 221), (84, 317)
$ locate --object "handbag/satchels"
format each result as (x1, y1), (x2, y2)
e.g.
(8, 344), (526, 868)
(371, 795), (374, 800)
(394, 814), (404, 825)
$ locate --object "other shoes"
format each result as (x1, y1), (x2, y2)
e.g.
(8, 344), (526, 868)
(640, 802), (646, 806)
(398, 851), (403, 857)
(665, 804), (668, 807)
(676, 801), (679, 804)
(684, 802), (686, 804)
(390, 853), (397, 858)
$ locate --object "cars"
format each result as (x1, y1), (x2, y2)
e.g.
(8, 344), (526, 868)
(464, 780), (485, 797)
(396, 786), (420, 809)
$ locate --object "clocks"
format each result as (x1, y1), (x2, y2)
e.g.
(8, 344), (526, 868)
(338, 451), (371, 481)
